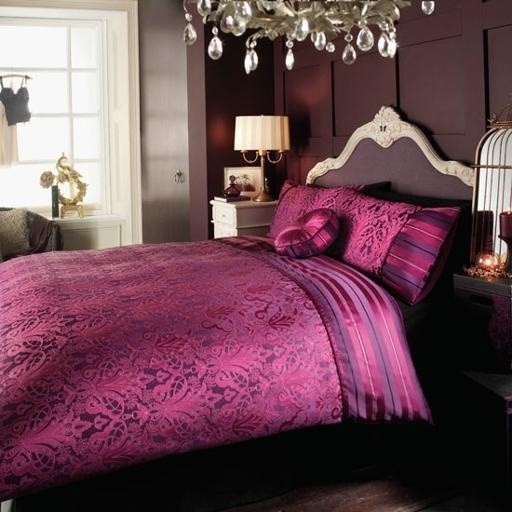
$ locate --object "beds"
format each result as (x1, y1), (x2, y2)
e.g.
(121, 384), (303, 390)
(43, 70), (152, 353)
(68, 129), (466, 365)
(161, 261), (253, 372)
(0, 105), (479, 512)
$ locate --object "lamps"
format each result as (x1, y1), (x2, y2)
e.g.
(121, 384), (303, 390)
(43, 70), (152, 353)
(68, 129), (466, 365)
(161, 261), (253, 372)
(183, 0), (435, 75)
(234, 116), (291, 203)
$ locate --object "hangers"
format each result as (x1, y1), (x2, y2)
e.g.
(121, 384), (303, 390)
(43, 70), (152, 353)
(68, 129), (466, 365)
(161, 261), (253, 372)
(0, 66), (31, 80)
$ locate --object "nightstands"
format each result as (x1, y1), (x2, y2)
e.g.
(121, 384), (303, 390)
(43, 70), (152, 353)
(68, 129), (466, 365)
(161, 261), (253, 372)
(210, 200), (278, 240)
(454, 274), (511, 372)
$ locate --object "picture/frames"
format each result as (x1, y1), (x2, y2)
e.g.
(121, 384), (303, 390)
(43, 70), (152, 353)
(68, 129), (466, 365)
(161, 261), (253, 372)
(224, 167), (265, 201)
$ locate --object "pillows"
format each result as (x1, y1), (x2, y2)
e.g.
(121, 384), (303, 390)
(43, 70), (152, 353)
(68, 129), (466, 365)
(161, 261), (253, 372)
(308, 181), (392, 195)
(274, 208), (341, 258)
(0, 207), (33, 256)
(269, 179), (461, 305)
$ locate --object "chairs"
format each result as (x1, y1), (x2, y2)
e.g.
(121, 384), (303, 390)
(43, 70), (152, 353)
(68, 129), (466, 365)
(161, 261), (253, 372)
(0, 207), (58, 261)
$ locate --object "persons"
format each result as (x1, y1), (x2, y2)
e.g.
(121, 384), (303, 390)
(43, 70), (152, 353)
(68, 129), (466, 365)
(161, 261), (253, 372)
(242, 174), (256, 192)
(236, 176), (243, 191)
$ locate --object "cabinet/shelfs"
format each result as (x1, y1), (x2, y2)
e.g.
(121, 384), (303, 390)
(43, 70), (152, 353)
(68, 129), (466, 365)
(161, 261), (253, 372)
(40, 212), (124, 249)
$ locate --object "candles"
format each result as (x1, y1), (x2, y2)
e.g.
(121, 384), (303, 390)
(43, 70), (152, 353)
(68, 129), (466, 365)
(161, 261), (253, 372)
(499, 210), (512, 236)
